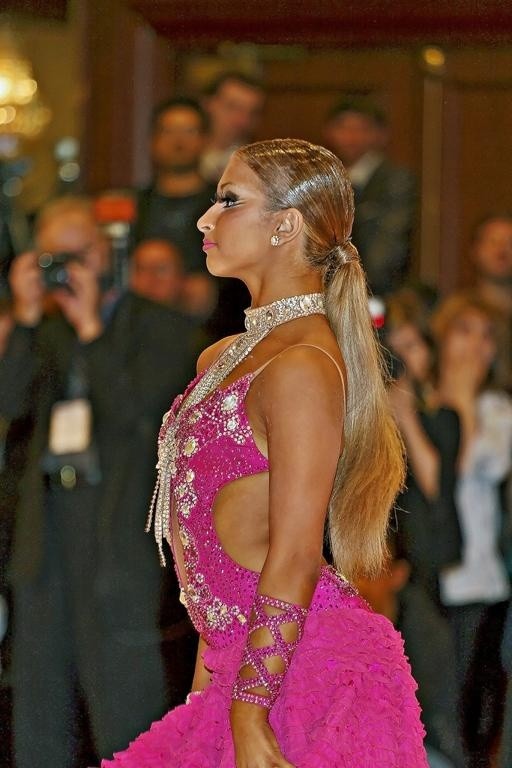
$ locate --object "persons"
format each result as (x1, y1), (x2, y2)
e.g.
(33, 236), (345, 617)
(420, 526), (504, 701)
(325, 91), (413, 289)
(368, 214), (512, 768)
(117, 60), (252, 339)
(0, 195), (211, 768)
(98, 137), (428, 768)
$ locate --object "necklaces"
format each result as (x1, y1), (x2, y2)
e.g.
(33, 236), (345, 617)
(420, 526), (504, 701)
(243, 293), (327, 333)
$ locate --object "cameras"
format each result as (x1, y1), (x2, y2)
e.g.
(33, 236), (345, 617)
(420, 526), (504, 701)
(42, 254), (81, 289)
